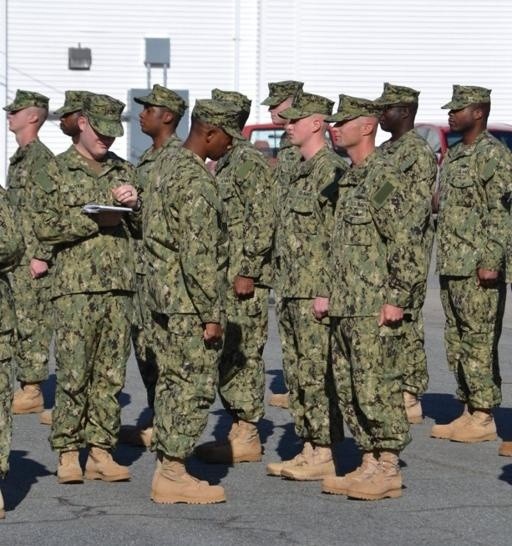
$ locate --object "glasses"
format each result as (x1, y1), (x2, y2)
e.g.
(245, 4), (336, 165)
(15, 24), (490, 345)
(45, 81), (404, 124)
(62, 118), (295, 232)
(383, 105), (408, 110)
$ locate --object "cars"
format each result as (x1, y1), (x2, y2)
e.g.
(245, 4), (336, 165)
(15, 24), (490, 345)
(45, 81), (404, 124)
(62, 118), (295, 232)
(416, 122), (512, 211)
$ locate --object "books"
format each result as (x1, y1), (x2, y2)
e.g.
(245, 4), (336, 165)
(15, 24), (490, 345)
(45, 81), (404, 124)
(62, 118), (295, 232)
(81, 203), (131, 215)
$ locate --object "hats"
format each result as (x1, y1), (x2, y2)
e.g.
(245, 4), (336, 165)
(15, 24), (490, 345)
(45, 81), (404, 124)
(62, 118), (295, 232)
(261, 81), (304, 106)
(54, 91), (96, 114)
(441, 85), (492, 110)
(278, 94), (335, 120)
(374, 83), (420, 106)
(324, 94), (383, 123)
(193, 99), (246, 141)
(134, 84), (189, 117)
(82, 95), (126, 136)
(2, 89), (48, 111)
(212, 89), (251, 114)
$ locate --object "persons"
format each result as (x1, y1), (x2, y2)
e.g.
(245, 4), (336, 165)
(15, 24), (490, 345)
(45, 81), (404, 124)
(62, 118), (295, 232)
(5, 89), (61, 411)
(267, 91), (338, 479)
(319, 93), (405, 501)
(0, 184), (26, 516)
(39, 90), (91, 426)
(432, 86), (512, 442)
(145, 101), (242, 508)
(373, 83), (436, 422)
(258, 80), (309, 410)
(197, 87), (277, 464)
(133, 86), (186, 449)
(40, 93), (140, 483)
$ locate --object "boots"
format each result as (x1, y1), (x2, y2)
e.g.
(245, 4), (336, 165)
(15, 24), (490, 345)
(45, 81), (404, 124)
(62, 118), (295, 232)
(403, 392), (424, 425)
(322, 451), (379, 495)
(149, 454), (209, 499)
(57, 451), (82, 484)
(40, 408), (52, 423)
(266, 440), (315, 476)
(347, 451), (403, 500)
(451, 409), (497, 441)
(13, 383), (44, 415)
(85, 448), (131, 482)
(199, 420), (262, 464)
(153, 455), (227, 505)
(268, 390), (289, 408)
(499, 440), (512, 456)
(430, 403), (474, 439)
(120, 407), (154, 446)
(280, 447), (337, 481)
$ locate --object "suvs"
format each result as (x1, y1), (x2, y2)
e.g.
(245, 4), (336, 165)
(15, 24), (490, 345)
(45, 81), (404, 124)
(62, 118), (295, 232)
(241, 121), (337, 164)
